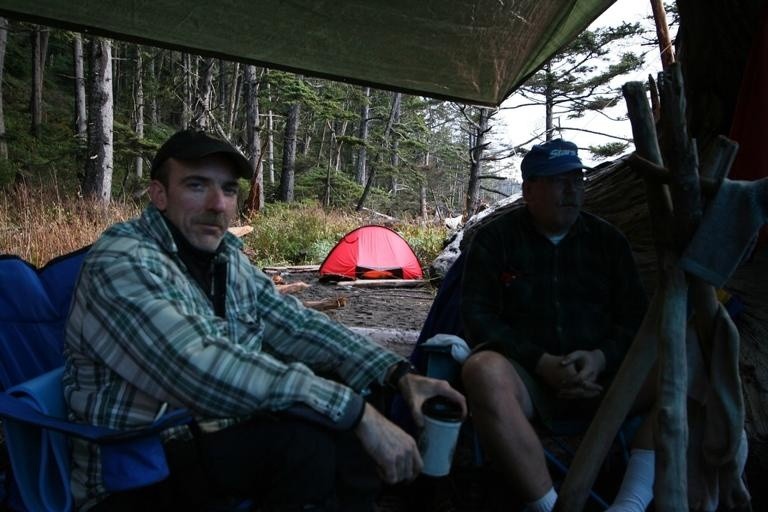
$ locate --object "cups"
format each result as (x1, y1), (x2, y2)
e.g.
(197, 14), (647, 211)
(414, 394), (463, 478)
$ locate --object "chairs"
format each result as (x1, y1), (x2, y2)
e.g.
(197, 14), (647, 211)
(424, 235), (661, 512)
(0, 240), (361, 512)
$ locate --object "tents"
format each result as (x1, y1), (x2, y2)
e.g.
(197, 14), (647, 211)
(317, 226), (425, 287)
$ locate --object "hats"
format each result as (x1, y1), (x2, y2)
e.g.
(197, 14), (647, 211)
(520, 138), (598, 182)
(149, 127), (257, 182)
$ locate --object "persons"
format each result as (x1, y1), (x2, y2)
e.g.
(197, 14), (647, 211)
(57, 129), (468, 511)
(407, 137), (659, 512)
